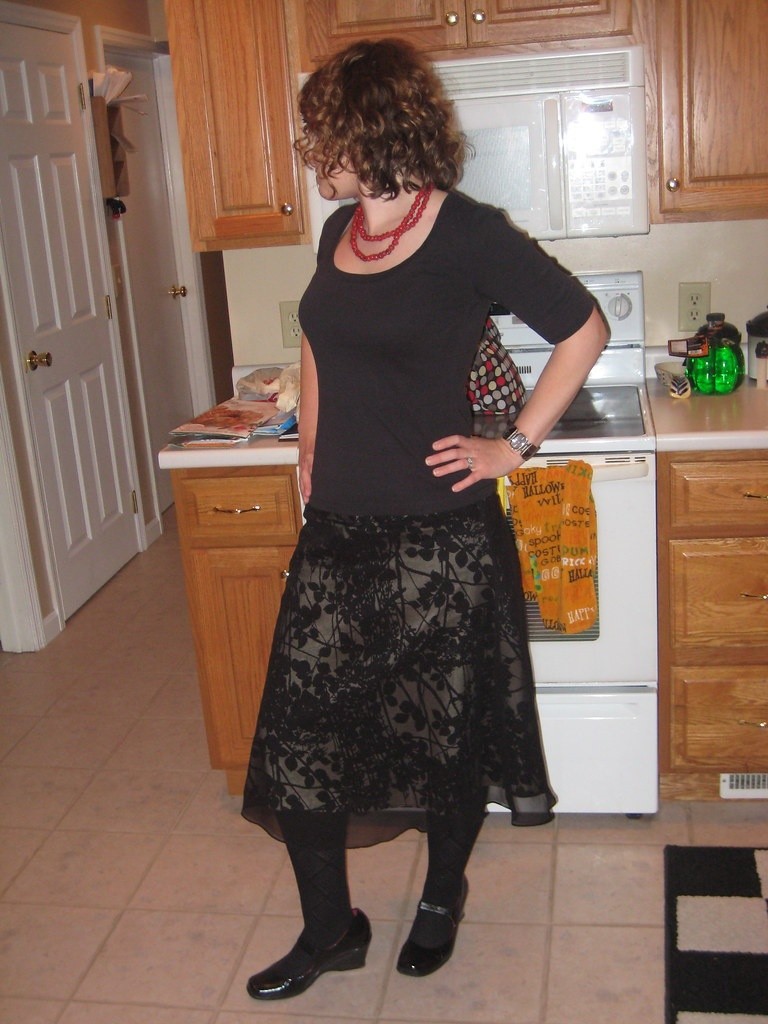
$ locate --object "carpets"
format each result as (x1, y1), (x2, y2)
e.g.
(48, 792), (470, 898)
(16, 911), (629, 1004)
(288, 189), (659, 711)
(662, 846), (767, 1024)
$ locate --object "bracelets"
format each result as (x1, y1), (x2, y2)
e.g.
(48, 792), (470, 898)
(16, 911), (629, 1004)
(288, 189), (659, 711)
(503, 423), (541, 462)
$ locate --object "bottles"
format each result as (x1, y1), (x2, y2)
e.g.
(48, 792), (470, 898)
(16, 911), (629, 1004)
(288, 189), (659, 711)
(686, 311), (745, 395)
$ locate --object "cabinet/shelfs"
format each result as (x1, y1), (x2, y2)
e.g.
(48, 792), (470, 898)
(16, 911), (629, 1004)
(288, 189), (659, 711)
(645, 1), (768, 226)
(171, 465), (299, 796)
(659, 451), (768, 801)
(164, 0), (305, 254)
(296, 0), (645, 75)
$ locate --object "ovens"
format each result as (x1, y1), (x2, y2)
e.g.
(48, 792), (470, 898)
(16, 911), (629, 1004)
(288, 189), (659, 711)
(290, 453), (660, 820)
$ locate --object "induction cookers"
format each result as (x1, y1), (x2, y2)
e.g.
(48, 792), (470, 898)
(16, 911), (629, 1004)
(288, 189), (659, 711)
(471, 271), (656, 451)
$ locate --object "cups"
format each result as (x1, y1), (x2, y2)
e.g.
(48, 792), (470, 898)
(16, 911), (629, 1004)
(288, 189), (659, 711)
(747, 306), (768, 381)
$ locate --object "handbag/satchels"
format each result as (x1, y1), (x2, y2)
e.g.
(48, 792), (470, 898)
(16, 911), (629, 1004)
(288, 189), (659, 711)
(466, 315), (525, 415)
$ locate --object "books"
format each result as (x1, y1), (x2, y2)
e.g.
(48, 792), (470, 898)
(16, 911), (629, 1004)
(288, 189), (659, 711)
(167, 396), (299, 448)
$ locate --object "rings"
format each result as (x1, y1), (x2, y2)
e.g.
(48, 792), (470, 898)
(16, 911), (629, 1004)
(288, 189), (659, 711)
(466, 457), (472, 469)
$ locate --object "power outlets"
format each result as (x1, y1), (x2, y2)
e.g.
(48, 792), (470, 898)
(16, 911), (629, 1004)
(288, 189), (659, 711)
(677, 281), (710, 333)
(279, 301), (303, 349)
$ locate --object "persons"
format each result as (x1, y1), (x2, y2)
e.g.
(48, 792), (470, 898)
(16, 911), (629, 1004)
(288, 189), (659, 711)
(240, 36), (613, 998)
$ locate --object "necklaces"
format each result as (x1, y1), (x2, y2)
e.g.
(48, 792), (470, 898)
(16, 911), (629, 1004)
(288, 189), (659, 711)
(348, 177), (434, 263)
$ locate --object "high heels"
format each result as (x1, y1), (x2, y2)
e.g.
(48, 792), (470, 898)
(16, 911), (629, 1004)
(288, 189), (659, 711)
(246, 907), (371, 1001)
(397, 873), (468, 976)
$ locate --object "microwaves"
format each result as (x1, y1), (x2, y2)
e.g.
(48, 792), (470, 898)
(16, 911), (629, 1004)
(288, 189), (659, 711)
(296, 45), (649, 253)
